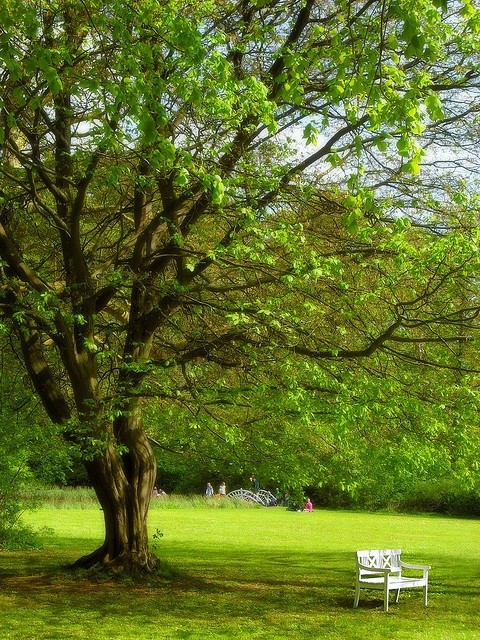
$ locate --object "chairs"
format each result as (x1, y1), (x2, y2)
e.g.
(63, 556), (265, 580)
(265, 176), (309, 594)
(353, 549), (432, 611)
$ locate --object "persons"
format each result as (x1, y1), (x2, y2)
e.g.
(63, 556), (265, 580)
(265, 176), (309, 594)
(218, 481), (227, 495)
(304, 498), (313, 512)
(152, 487), (158, 497)
(205, 483), (213, 495)
